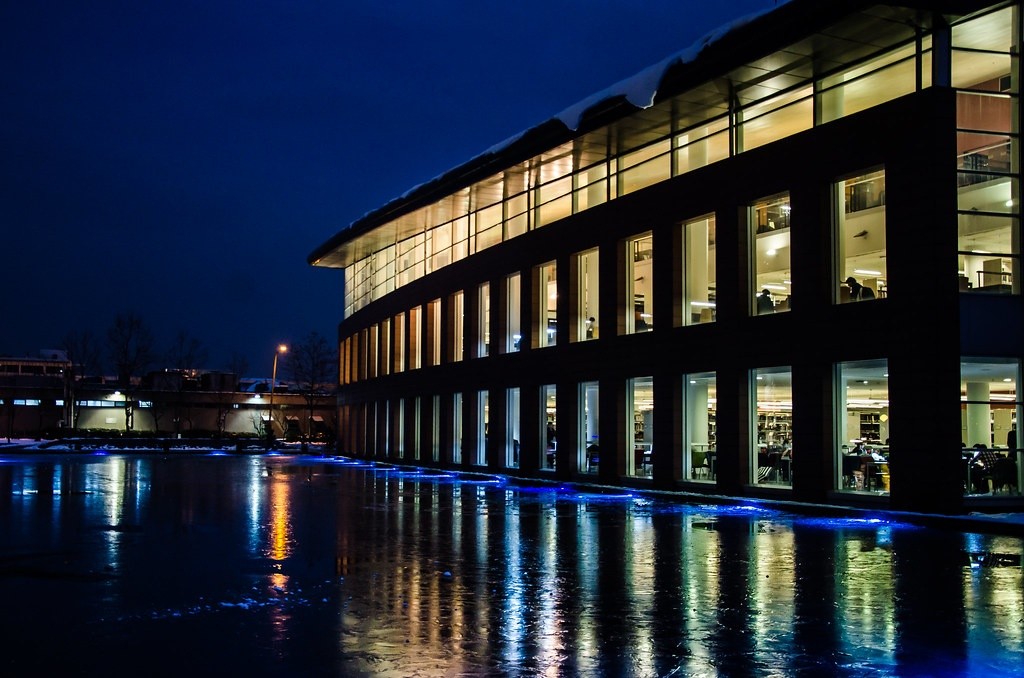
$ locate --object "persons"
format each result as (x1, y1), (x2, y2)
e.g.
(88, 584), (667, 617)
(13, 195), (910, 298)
(585, 315), (595, 332)
(758, 289), (773, 312)
(846, 276), (864, 299)
(978, 445), (998, 494)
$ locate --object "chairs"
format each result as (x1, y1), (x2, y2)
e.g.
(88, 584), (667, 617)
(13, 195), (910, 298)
(546, 435), (1018, 494)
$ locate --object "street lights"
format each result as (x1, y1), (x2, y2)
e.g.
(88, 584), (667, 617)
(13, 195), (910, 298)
(266, 344), (287, 436)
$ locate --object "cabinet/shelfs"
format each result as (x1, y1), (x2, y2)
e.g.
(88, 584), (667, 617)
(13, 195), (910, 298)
(859, 413), (881, 440)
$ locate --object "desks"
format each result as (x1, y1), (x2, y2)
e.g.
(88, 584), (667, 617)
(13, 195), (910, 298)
(635, 443), (651, 449)
(691, 442), (709, 452)
